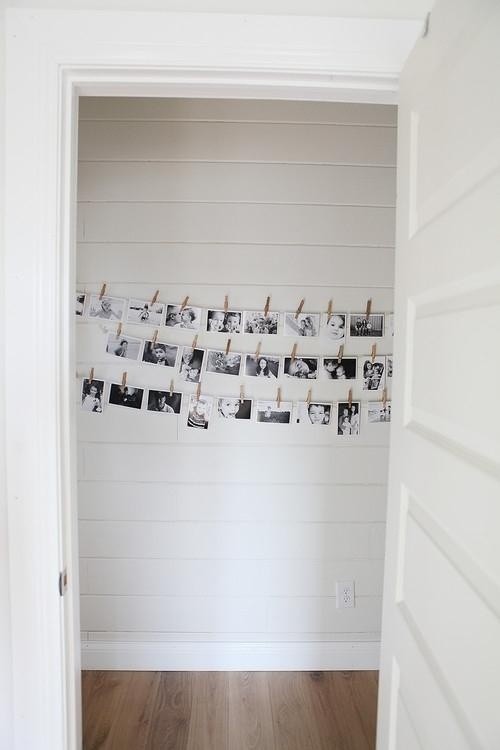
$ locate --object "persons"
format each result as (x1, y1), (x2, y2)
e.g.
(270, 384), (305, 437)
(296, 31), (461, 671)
(74, 294), (392, 437)
